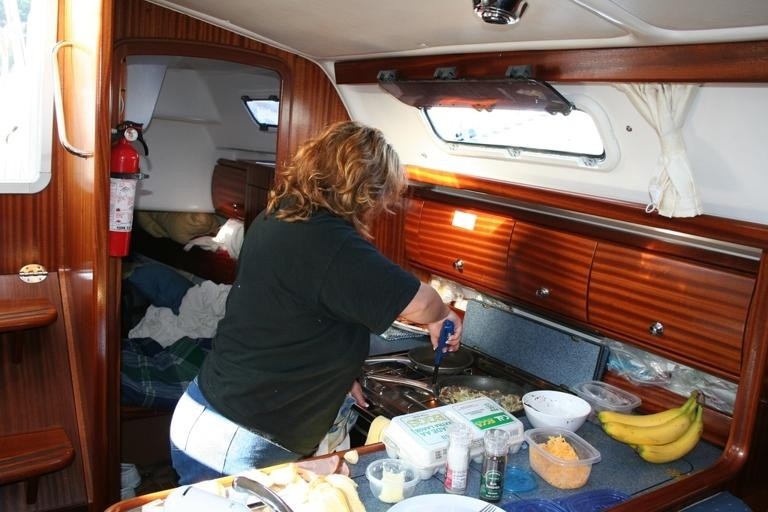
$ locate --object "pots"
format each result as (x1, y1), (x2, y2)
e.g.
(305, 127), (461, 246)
(364, 371), (533, 415)
(363, 343), (480, 376)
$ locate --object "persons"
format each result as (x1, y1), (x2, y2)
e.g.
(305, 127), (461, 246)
(164, 123), (462, 489)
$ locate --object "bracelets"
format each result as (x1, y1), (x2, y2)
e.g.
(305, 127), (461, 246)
(524, 390), (594, 432)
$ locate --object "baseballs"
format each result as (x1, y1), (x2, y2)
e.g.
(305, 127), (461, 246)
(109, 121), (149, 257)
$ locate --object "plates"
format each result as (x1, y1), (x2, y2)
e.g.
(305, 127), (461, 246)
(1, 298), (76, 505)
(212, 158), (276, 231)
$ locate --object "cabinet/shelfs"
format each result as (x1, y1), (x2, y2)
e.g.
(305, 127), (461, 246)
(402, 178), (760, 390)
(212, 158), (276, 231)
(442, 429), (471, 495)
(477, 427), (511, 503)
(1, 298), (76, 505)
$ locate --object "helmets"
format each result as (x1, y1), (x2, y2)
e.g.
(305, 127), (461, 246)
(385, 492), (509, 512)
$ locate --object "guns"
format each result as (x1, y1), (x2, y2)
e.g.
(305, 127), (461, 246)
(599, 391), (705, 463)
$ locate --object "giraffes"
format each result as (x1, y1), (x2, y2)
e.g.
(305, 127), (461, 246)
(356, 333), (586, 434)
